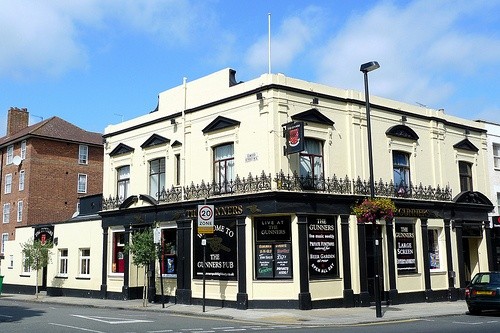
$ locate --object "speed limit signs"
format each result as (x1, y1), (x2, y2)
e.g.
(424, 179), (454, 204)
(198, 205), (214, 234)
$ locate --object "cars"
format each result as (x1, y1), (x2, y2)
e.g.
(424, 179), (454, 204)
(465, 272), (500, 315)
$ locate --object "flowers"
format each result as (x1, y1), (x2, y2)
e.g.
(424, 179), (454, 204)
(349, 195), (399, 224)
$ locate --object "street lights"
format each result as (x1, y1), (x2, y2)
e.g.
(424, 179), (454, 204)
(361, 62), (384, 318)
